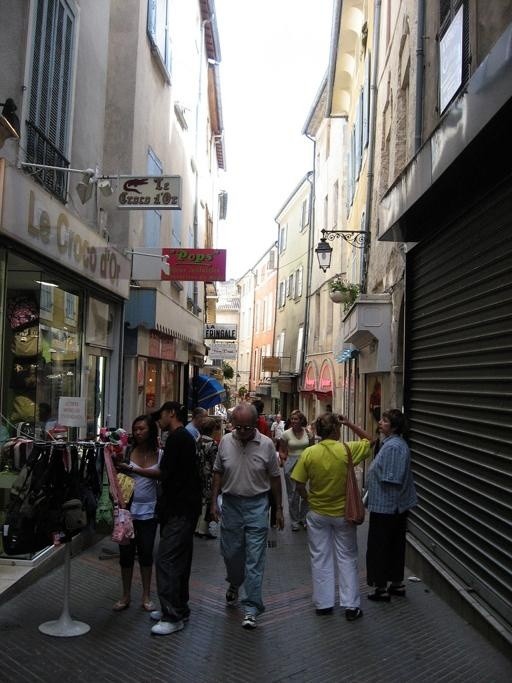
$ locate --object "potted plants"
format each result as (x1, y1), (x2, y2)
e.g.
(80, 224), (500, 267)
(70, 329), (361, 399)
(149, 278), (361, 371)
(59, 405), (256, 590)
(327, 275), (362, 315)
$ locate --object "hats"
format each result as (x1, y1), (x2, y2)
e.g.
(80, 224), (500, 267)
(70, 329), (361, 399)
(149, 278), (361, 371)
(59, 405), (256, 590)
(149, 400), (188, 422)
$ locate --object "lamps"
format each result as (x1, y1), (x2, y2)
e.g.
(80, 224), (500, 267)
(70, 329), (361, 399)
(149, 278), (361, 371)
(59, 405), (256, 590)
(21, 160), (95, 207)
(1, 96), (22, 138)
(315, 227), (370, 274)
(125, 248), (171, 277)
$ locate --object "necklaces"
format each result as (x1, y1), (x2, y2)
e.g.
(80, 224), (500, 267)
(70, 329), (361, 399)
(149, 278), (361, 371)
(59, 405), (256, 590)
(138, 449), (155, 464)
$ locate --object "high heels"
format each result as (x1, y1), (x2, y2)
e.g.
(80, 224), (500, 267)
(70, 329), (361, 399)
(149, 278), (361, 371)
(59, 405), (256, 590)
(198, 532), (217, 540)
(194, 531), (199, 536)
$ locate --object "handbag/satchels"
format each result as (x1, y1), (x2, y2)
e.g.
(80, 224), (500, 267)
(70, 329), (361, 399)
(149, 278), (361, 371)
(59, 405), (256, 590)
(345, 467), (365, 525)
(111, 509), (135, 546)
(3, 445), (104, 555)
(110, 472), (133, 510)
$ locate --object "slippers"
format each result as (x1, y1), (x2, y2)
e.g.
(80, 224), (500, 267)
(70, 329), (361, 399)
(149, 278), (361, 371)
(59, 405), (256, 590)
(113, 600), (132, 610)
(143, 600), (157, 611)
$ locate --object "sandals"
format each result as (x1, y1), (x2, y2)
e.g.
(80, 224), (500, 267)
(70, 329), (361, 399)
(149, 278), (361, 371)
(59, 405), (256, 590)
(367, 590), (390, 603)
(387, 584), (406, 597)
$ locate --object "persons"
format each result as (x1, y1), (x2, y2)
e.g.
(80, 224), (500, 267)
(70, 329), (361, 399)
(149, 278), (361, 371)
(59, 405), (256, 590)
(113, 401), (202, 636)
(109, 398), (315, 541)
(208, 401), (285, 631)
(364, 408), (419, 602)
(108, 413), (165, 613)
(289, 411), (378, 623)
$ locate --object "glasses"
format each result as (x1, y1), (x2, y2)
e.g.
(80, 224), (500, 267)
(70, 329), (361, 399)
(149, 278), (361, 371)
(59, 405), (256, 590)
(233, 425), (252, 431)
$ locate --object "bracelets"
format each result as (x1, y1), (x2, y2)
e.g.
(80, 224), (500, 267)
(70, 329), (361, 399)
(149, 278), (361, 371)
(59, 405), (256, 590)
(129, 466), (134, 474)
(275, 504), (284, 511)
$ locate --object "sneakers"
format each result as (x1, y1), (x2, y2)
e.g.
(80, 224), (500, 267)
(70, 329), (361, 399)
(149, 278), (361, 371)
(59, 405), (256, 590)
(292, 522), (299, 531)
(316, 607), (333, 615)
(301, 521), (307, 529)
(242, 604), (260, 628)
(345, 606), (362, 620)
(152, 623), (185, 634)
(151, 609), (189, 623)
(226, 582), (243, 605)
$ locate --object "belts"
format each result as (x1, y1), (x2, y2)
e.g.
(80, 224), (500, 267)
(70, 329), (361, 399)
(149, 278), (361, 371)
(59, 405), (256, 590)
(224, 492), (265, 501)
